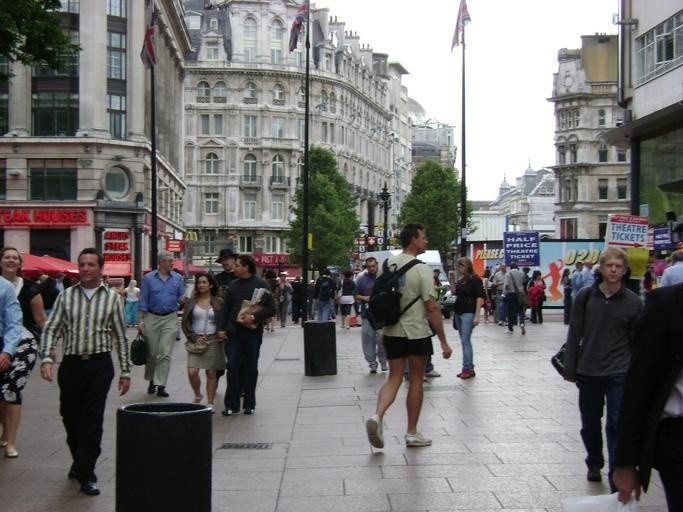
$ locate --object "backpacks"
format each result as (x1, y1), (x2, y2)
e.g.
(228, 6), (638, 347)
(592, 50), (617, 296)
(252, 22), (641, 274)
(319, 276), (331, 297)
(368, 259), (426, 326)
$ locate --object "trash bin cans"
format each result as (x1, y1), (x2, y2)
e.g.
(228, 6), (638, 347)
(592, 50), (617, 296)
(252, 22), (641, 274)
(303, 321), (337, 376)
(116, 401), (212, 512)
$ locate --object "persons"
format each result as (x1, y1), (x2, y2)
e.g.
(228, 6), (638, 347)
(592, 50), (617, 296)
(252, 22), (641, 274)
(567, 242), (682, 512)
(447, 259), (495, 376)
(37, 274), (74, 318)
(366, 225), (472, 450)
(2, 246), (50, 457)
(432, 263), (548, 334)
(419, 334), (443, 381)
(561, 260), (602, 326)
(264, 265), (366, 329)
(120, 249), (271, 416)
(36, 245), (132, 495)
(355, 255), (394, 374)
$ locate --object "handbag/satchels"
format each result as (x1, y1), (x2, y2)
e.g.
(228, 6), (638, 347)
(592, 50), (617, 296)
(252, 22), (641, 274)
(184, 335), (210, 354)
(131, 331), (146, 365)
(517, 293), (529, 307)
(453, 312), (458, 330)
(551, 342), (583, 382)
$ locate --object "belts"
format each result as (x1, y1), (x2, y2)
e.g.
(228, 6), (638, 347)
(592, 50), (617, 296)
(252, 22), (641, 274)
(69, 351), (110, 361)
(147, 309), (175, 316)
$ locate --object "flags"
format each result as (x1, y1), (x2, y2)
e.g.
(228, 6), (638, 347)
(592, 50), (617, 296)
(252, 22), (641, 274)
(288, 1), (313, 53)
(136, 12), (162, 67)
(449, 0), (472, 51)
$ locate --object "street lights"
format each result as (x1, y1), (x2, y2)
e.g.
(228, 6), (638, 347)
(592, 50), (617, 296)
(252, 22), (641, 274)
(378, 180), (392, 251)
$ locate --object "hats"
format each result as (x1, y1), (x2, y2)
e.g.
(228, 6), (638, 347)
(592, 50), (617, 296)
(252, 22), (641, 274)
(216, 248), (238, 262)
(433, 268), (441, 273)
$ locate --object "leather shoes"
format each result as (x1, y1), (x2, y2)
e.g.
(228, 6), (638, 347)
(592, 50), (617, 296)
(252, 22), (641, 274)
(68, 464), (101, 495)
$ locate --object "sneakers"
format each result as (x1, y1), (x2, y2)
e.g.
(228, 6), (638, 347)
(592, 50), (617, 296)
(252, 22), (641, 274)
(381, 362), (387, 371)
(587, 465), (601, 481)
(4, 448), (18, 457)
(405, 433), (432, 447)
(0, 440), (8, 446)
(370, 365), (376, 373)
(145, 383), (254, 416)
(424, 371), (440, 377)
(505, 330), (513, 334)
(366, 414), (384, 448)
(520, 323), (525, 334)
